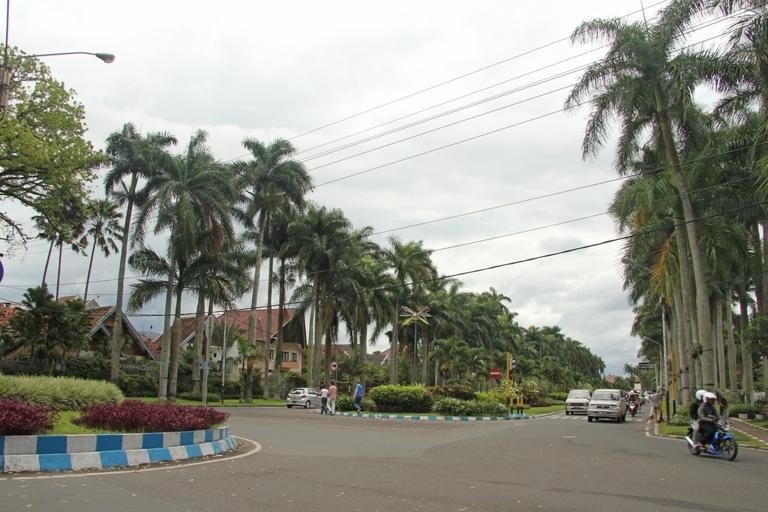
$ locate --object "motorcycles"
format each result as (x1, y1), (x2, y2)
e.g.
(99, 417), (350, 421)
(626, 389), (645, 417)
(685, 418), (737, 461)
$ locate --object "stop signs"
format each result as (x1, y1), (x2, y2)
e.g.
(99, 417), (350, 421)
(490, 368), (501, 379)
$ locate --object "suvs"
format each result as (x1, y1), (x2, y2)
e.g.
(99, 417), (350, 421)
(566, 389), (592, 415)
(588, 389), (627, 423)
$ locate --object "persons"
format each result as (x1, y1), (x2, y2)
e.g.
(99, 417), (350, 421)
(351, 379), (364, 417)
(327, 380), (337, 416)
(627, 389), (641, 412)
(649, 388), (662, 423)
(315, 385), (328, 414)
(689, 389), (708, 443)
(698, 391), (721, 455)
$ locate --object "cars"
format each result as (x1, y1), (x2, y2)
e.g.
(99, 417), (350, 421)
(644, 391), (655, 400)
(286, 388), (322, 409)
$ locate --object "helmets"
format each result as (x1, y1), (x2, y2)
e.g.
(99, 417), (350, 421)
(695, 389), (706, 401)
(703, 392), (716, 404)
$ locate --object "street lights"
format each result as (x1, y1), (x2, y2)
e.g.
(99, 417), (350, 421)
(0, 52), (115, 120)
(622, 256), (670, 422)
